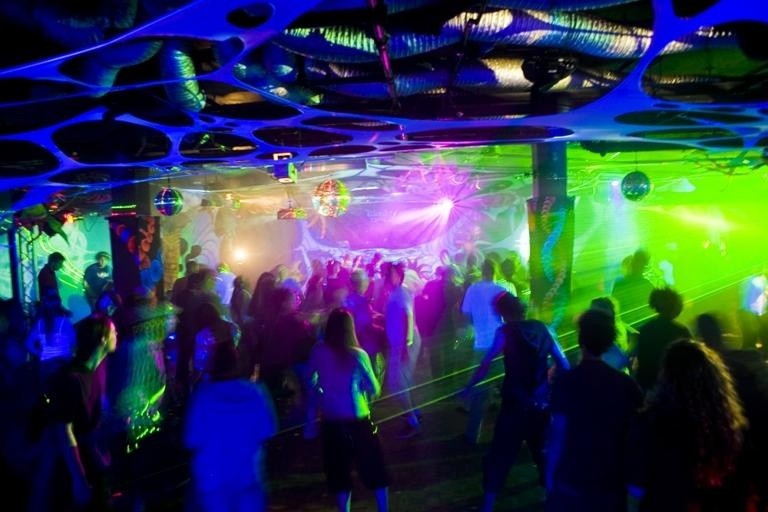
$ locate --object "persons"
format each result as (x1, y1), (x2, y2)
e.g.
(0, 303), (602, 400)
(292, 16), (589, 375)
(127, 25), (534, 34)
(1, 237), (768, 511)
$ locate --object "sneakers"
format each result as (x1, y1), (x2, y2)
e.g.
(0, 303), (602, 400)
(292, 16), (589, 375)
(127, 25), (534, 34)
(394, 415), (423, 439)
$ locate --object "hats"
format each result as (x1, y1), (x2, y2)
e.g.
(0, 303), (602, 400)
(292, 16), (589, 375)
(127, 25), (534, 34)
(96, 252), (111, 260)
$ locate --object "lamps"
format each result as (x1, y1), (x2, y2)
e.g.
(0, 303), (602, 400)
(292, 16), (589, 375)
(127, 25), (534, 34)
(434, 194), (454, 215)
(670, 180), (694, 194)
(276, 187), (307, 220)
(620, 152), (649, 203)
(154, 172), (183, 216)
(311, 180), (348, 218)
(199, 175), (241, 209)
